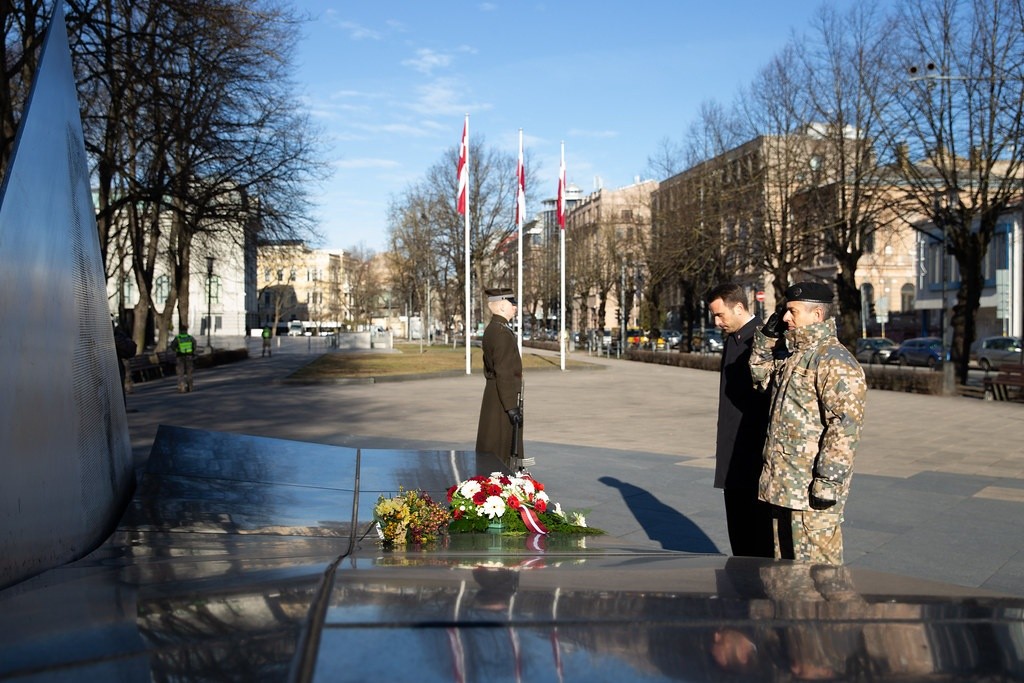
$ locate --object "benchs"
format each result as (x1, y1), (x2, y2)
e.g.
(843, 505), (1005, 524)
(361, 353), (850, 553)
(982, 363), (1024, 400)
(156, 351), (176, 376)
(128, 354), (162, 383)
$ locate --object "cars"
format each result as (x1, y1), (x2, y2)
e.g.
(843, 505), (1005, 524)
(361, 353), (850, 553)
(897, 338), (944, 367)
(856, 338), (898, 364)
(288, 323), (303, 335)
(976, 337), (1024, 371)
(546, 330), (558, 340)
(589, 328), (612, 347)
(574, 332), (580, 343)
(660, 330), (682, 348)
(644, 334), (666, 350)
(153, 328), (174, 346)
(691, 328), (725, 352)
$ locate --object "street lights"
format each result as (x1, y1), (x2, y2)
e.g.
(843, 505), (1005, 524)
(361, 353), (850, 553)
(118, 247), (125, 322)
(204, 256), (216, 353)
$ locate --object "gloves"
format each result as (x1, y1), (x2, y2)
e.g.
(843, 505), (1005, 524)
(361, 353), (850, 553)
(507, 408), (523, 425)
(809, 493), (837, 510)
(761, 306), (789, 338)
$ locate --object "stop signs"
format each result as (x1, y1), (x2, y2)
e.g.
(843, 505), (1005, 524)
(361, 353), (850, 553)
(756, 291), (766, 302)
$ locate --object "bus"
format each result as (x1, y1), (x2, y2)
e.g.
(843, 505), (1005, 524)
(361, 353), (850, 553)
(304, 326), (341, 336)
(611, 326), (644, 349)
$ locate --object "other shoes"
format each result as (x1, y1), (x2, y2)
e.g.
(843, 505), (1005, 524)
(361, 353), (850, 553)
(262, 355), (272, 358)
(180, 386), (193, 393)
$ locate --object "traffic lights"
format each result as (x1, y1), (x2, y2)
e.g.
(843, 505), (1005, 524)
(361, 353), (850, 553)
(615, 309), (619, 320)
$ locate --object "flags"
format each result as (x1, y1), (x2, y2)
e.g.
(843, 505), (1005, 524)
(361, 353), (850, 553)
(508, 624), (523, 683)
(447, 625), (467, 683)
(515, 142), (529, 225)
(456, 116), (469, 214)
(550, 625), (565, 683)
(555, 149), (564, 232)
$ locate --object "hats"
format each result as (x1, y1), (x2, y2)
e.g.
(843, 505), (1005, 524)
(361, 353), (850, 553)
(484, 287), (518, 304)
(784, 281), (835, 303)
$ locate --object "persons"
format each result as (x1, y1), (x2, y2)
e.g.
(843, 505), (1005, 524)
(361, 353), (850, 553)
(109, 313), (139, 414)
(475, 286), (527, 472)
(711, 623), (778, 683)
(790, 619), (870, 683)
(262, 325), (273, 357)
(749, 282), (869, 567)
(171, 325), (197, 394)
(705, 282), (792, 558)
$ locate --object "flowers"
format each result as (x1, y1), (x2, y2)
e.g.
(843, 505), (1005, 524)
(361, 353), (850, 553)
(371, 465), (588, 553)
